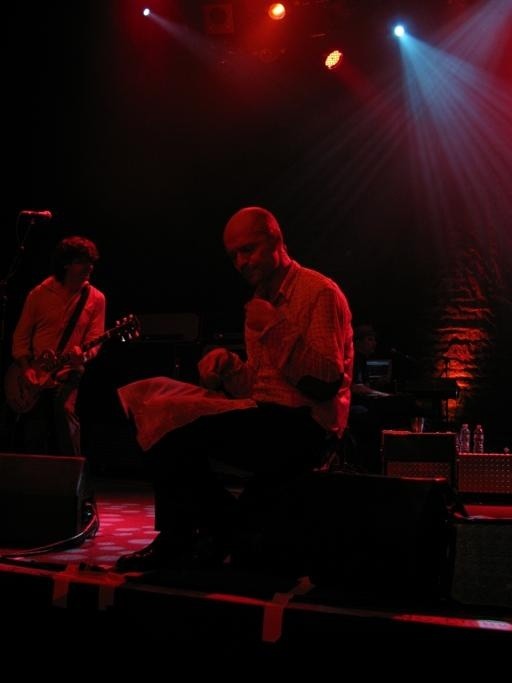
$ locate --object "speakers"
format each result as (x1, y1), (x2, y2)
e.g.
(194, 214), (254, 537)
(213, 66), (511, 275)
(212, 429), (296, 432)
(313, 471), (458, 615)
(0, 454), (95, 549)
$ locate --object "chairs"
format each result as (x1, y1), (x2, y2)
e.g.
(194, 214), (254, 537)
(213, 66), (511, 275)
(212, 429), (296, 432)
(1, 450), (102, 561)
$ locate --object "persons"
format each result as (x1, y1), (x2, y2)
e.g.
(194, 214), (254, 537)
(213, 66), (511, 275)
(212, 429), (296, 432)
(8, 237), (107, 459)
(351, 323), (415, 468)
(111, 205), (356, 603)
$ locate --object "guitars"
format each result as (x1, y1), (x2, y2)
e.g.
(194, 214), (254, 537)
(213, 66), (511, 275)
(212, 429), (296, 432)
(2, 313), (142, 417)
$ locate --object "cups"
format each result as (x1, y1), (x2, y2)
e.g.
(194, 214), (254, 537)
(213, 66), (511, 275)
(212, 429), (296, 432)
(415, 417), (424, 433)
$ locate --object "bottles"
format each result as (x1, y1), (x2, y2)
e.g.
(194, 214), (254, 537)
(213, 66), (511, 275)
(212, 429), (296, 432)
(460, 424), (484, 453)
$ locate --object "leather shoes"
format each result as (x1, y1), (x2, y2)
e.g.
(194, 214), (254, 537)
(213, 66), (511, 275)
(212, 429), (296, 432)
(115, 531), (161, 569)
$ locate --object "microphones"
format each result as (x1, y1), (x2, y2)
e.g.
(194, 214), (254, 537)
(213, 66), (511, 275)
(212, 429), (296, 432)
(392, 348), (416, 361)
